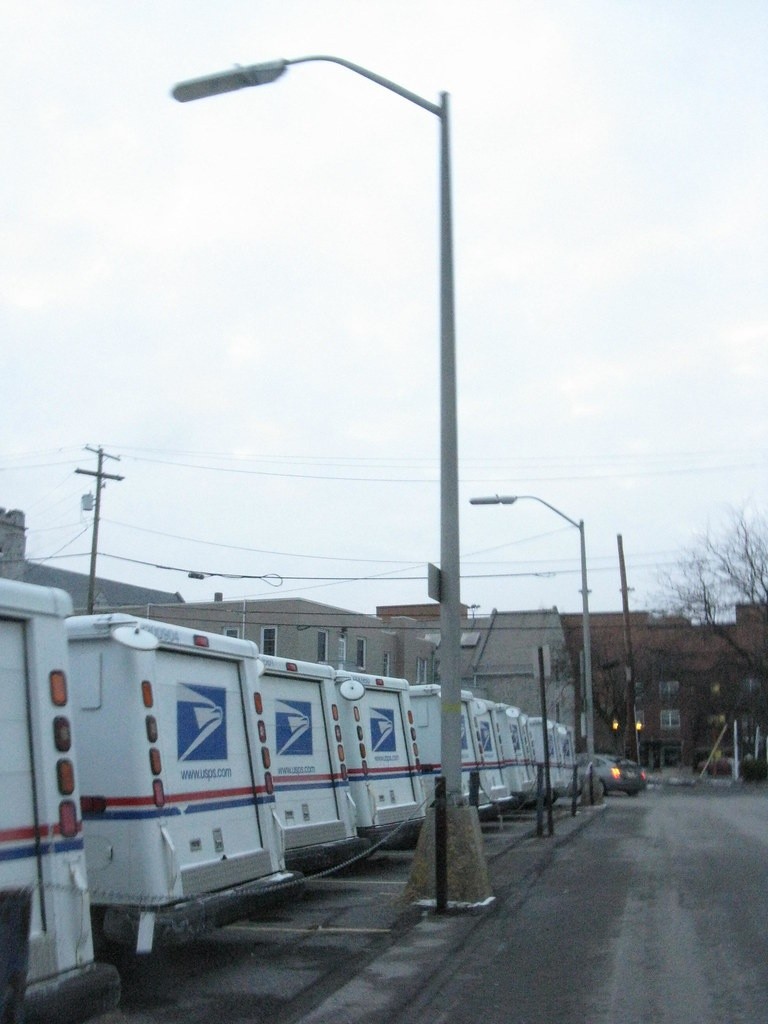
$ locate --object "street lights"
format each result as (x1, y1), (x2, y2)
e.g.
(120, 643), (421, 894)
(170, 53), (491, 907)
(470, 496), (596, 805)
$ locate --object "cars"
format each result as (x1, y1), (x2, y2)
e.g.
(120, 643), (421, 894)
(597, 754), (648, 798)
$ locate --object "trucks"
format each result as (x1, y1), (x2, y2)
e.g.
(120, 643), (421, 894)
(0, 579), (578, 1024)
